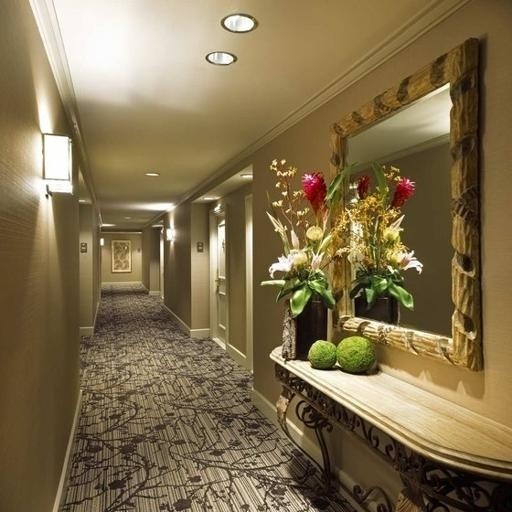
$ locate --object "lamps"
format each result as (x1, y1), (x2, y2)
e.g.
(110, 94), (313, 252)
(42, 133), (73, 194)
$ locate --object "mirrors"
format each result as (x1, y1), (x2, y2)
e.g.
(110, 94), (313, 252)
(330, 36), (482, 371)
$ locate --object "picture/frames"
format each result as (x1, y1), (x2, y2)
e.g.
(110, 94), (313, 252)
(111, 240), (132, 272)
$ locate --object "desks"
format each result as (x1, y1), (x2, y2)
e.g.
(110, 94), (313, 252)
(270, 346), (511, 511)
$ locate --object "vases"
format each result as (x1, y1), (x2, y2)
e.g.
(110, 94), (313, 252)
(295, 302), (327, 360)
(356, 297), (397, 325)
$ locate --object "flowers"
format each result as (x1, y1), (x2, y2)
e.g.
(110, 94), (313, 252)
(351, 161), (423, 309)
(261, 157), (359, 319)
